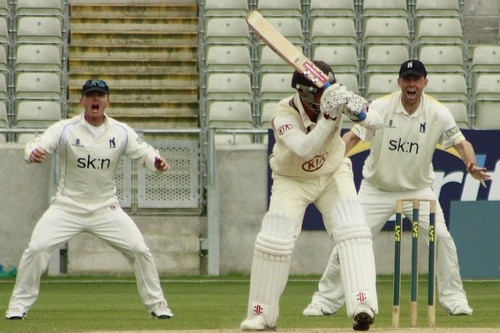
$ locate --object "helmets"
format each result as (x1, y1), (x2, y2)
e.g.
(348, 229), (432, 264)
(292, 61), (336, 92)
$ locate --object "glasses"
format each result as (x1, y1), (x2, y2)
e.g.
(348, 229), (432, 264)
(84, 80), (106, 88)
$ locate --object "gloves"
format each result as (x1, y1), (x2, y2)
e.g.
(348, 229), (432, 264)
(320, 83), (369, 121)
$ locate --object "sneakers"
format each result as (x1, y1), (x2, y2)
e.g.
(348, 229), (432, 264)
(150, 303), (174, 318)
(5, 308), (27, 319)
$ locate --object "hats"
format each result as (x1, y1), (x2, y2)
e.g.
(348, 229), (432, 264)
(82, 79), (109, 93)
(399, 60), (426, 76)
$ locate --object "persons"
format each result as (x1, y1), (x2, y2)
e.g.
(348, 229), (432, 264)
(5, 79), (174, 320)
(241, 60), (382, 331)
(304, 60), (492, 317)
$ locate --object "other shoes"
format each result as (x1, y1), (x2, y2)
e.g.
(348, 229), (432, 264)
(353, 303), (374, 331)
(451, 304), (473, 315)
(241, 314), (277, 332)
(303, 303), (324, 315)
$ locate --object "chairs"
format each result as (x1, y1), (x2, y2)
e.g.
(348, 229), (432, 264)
(0, 0), (69, 156)
(198, 0), (500, 147)
